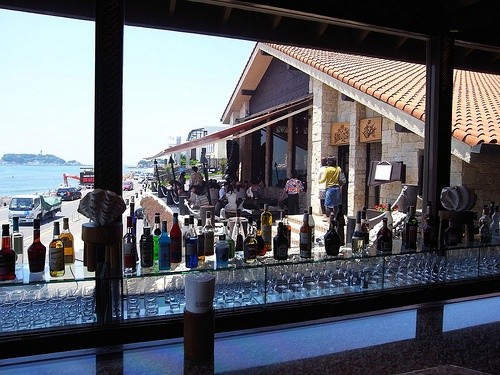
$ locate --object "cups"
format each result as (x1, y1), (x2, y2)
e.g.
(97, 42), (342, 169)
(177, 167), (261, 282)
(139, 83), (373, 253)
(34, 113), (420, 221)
(122, 269), (254, 319)
(0, 285), (96, 331)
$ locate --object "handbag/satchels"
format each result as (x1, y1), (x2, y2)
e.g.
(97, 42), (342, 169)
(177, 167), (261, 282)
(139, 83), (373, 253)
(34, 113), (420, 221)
(339, 168), (347, 185)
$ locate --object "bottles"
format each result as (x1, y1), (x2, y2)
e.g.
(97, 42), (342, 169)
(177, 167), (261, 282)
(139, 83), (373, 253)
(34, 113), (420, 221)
(377, 203), (393, 254)
(422, 201), (500, 246)
(274, 208), (291, 260)
(0, 224), (17, 281)
(401, 205), (418, 250)
(122, 203), (273, 270)
(48, 221), (65, 277)
(299, 205), (314, 257)
(60, 218), (75, 263)
(27, 219), (46, 273)
(324, 203), (345, 257)
(352, 206), (369, 254)
(11, 217), (24, 265)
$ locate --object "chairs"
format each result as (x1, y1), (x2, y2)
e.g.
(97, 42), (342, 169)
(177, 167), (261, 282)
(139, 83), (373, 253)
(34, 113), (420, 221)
(158, 186), (254, 229)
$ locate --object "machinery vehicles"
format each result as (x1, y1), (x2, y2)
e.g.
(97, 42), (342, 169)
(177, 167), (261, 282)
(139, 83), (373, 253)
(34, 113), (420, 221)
(63, 167), (95, 189)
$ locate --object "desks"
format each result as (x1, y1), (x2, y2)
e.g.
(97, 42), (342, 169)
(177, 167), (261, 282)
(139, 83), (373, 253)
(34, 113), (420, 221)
(260, 206), (283, 220)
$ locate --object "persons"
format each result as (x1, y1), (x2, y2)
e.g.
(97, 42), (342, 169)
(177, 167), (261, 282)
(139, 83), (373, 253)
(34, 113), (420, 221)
(189, 175), (265, 214)
(317, 157), (342, 222)
(285, 171), (304, 215)
(180, 171), (186, 190)
(190, 166), (203, 186)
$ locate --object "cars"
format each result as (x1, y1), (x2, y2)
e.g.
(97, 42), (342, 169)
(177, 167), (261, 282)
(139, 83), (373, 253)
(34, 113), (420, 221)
(58, 187), (82, 201)
(123, 181), (133, 191)
(133, 172), (154, 183)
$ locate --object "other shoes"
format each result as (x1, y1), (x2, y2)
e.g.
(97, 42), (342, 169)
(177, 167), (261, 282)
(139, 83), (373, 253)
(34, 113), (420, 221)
(323, 218), (329, 221)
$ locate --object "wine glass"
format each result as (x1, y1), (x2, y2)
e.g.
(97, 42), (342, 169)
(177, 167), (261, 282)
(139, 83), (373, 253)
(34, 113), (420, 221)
(254, 245), (500, 302)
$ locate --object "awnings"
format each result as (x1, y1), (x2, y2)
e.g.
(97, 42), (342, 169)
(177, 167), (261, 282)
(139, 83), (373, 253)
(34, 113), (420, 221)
(164, 92), (313, 155)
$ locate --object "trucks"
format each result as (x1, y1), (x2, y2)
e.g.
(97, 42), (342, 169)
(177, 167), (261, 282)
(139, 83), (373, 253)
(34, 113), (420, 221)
(4, 195), (62, 226)
